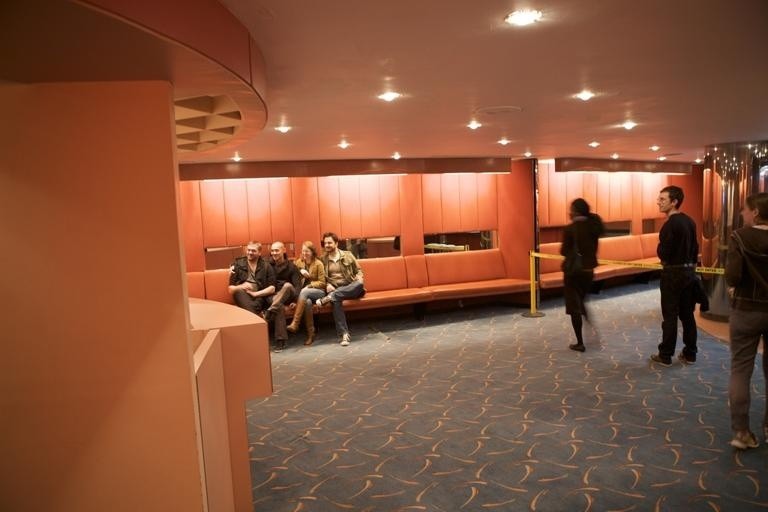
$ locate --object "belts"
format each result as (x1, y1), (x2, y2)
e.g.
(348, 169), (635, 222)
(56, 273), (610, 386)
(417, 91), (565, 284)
(663, 262), (696, 270)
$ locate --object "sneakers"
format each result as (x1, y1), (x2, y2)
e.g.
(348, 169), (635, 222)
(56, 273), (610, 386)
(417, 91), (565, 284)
(677, 351), (693, 363)
(650, 352), (672, 368)
(261, 310), (275, 323)
(273, 340), (288, 353)
(339, 332), (352, 346)
(316, 295), (332, 308)
(731, 433), (759, 451)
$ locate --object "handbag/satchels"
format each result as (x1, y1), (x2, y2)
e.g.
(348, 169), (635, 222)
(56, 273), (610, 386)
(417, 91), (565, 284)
(560, 224), (584, 271)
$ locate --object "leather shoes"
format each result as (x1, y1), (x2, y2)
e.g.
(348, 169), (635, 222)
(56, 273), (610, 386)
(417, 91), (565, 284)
(569, 343), (586, 353)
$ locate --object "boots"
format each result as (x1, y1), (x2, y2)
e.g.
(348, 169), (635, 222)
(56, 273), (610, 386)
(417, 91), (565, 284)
(287, 299), (306, 333)
(304, 311), (315, 345)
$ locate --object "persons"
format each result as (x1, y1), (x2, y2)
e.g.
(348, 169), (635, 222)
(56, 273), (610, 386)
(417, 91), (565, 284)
(227, 241), (276, 315)
(650, 186), (708, 367)
(724, 192), (768, 450)
(261, 241), (302, 353)
(287, 240), (326, 345)
(560, 198), (602, 353)
(320, 232), (365, 346)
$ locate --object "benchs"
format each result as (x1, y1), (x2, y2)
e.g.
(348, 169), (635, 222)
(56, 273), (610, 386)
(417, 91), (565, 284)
(537, 231), (662, 301)
(185, 238), (539, 324)
